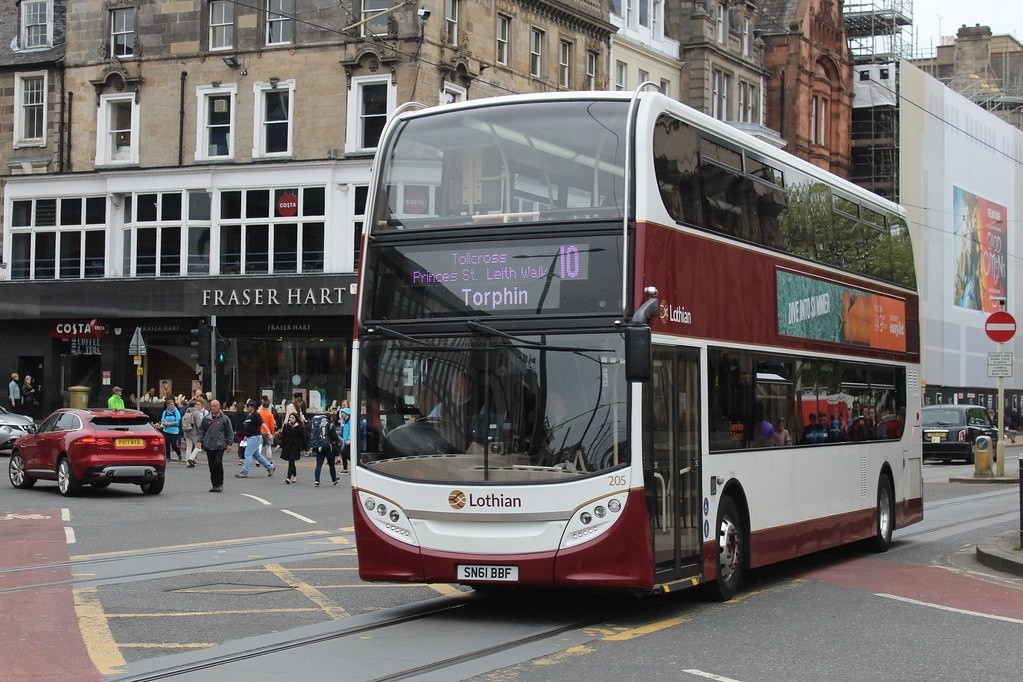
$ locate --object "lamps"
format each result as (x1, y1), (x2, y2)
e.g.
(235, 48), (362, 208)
(223, 56), (241, 68)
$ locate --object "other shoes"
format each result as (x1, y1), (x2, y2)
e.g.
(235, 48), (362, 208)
(333, 477), (340, 486)
(208, 485), (224, 493)
(314, 481), (319, 487)
(166, 458), (171, 463)
(267, 467), (275, 477)
(186, 459), (195, 469)
(286, 476), (297, 484)
(178, 455), (183, 462)
(235, 472), (249, 478)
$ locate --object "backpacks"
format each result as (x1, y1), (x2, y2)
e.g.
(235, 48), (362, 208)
(182, 409), (196, 432)
(311, 415), (333, 451)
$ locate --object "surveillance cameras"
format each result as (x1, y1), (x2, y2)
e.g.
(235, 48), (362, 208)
(417, 9), (431, 18)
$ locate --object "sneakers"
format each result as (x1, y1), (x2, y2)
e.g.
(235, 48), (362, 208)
(339, 470), (348, 474)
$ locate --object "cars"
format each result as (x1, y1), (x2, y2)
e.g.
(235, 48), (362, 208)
(921, 404), (998, 464)
(0, 406), (37, 450)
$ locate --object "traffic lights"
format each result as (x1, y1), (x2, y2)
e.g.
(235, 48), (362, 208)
(215, 339), (229, 366)
(190, 322), (209, 367)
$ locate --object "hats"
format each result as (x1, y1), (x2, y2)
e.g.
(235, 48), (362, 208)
(113, 386), (123, 391)
(247, 401), (258, 407)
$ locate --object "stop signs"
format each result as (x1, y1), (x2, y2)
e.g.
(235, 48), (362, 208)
(984, 311), (1017, 344)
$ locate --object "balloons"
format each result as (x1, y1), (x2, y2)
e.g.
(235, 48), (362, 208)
(760, 421), (774, 438)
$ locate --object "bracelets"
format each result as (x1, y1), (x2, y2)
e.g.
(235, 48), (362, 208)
(267, 433), (270, 435)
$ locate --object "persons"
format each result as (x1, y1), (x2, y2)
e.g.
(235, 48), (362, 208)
(989, 410), (998, 428)
(771, 416), (792, 447)
(230, 392), (366, 488)
(193, 381), (202, 397)
(408, 372), (485, 455)
(1007, 408), (1021, 444)
(22, 375), (35, 420)
(162, 381), (171, 397)
(197, 400), (234, 492)
(141, 388), (212, 468)
(9, 373), (20, 415)
(799, 406), (887, 445)
(107, 387), (125, 409)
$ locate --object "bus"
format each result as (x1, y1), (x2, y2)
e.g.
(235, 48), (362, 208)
(347, 80), (923, 602)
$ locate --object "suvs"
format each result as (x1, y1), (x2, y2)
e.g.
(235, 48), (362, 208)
(8, 408), (167, 496)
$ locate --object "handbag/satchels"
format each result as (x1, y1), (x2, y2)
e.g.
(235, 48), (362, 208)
(271, 403), (278, 420)
